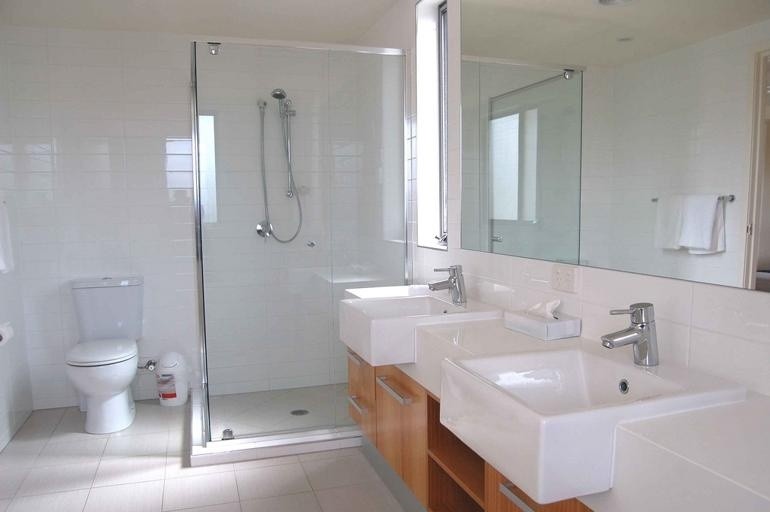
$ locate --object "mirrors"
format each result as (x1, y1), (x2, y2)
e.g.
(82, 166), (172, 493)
(458, 1), (770, 294)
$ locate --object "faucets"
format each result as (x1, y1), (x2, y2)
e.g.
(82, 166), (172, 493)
(428, 265), (467, 304)
(601, 303), (659, 366)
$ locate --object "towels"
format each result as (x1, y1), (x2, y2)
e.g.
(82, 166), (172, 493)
(655, 192), (727, 254)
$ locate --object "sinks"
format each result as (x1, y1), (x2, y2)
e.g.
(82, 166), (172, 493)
(338, 293), (504, 367)
(439, 347), (746, 505)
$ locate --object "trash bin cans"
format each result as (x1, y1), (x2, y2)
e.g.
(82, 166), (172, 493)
(155, 351), (189, 407)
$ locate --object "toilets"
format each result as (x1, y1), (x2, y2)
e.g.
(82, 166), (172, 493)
(65, 276), (145, 435)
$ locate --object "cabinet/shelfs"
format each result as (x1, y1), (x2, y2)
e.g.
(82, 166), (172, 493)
(347, 346), (377, 450)
(427, 391), (485, 512)
(485, 460), (593, 512)
(372, 364), (429, 512)
